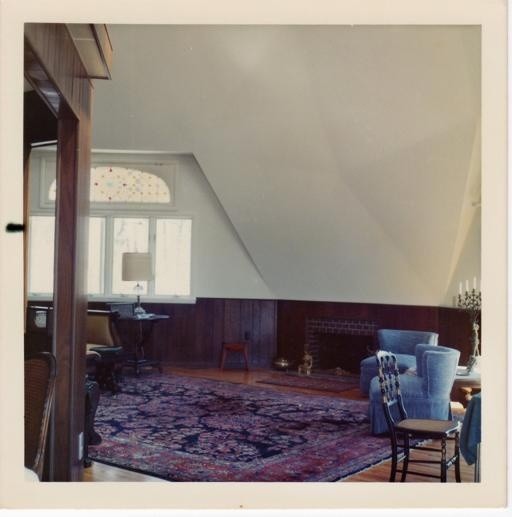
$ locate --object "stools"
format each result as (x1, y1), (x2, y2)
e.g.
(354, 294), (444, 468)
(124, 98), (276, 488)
(220, 341), (249, 374)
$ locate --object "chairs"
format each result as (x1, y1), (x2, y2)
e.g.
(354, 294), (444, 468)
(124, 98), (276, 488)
(24, 350), (58, 482)
(25, 301), (127, 397)
(373, 349), (463, 482)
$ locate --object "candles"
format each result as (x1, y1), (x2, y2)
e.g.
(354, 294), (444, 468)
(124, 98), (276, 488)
(465, 280), (468, 292)
(473, 276), (476, 288)
(459, 281), (463, 294)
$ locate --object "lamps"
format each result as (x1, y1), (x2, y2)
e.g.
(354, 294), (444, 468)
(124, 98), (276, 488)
(121, 250), (156, 314)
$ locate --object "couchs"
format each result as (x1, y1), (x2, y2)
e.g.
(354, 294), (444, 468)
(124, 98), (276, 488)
(368, 343), (462, 440)
(358, 327), (440, 396)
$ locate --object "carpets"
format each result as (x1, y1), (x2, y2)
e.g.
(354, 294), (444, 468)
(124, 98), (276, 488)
(255, 371), (358, 394)
(80, 368), (434, 482)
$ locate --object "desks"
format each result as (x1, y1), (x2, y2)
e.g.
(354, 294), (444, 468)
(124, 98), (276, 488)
(111, 311), (170, 375)
